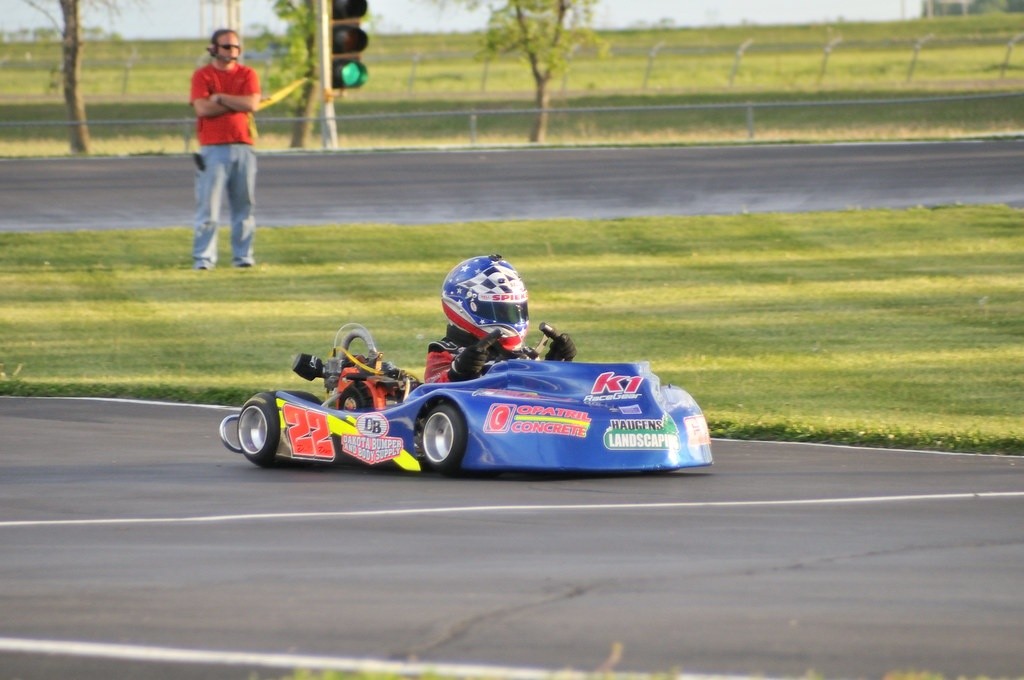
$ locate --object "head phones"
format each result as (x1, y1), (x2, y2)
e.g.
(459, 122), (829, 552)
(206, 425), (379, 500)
(207, 43), (242, 57)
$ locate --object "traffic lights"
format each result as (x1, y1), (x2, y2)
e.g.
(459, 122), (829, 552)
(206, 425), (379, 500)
(331, 0), (371, 92)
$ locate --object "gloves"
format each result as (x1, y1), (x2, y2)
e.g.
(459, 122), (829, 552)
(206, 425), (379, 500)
(544, 333), (577, 361)
(446, 345), (487, 382)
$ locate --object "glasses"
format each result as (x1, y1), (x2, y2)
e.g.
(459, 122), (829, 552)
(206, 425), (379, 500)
(218, 45), (239, 50)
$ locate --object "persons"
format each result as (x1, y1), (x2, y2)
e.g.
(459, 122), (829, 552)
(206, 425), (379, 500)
(424, 256), (577, 383)
(190, 30), (261, 269)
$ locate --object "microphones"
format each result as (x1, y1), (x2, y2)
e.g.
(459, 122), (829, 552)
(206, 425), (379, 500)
(215, 54), (230, 63)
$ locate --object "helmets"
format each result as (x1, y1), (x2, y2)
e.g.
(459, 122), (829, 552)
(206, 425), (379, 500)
(441, 254), (529, 355)
